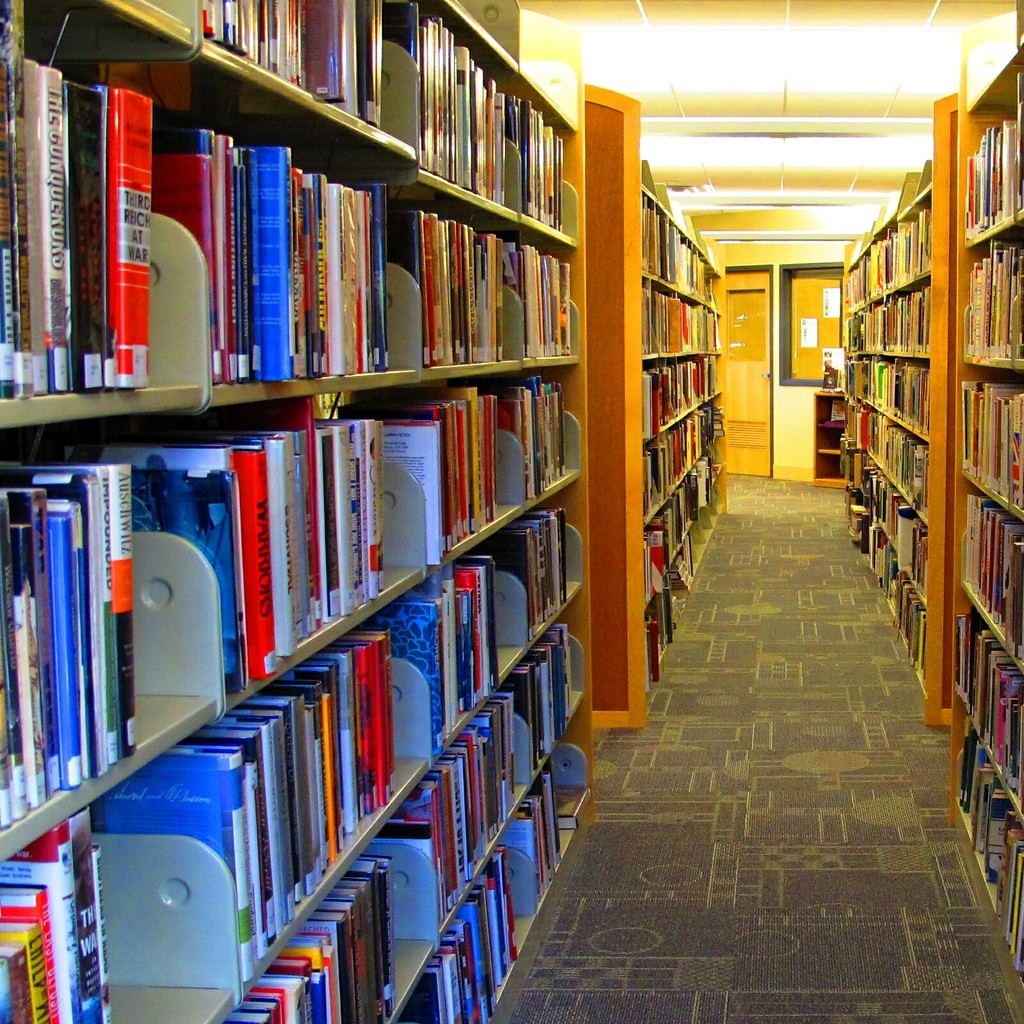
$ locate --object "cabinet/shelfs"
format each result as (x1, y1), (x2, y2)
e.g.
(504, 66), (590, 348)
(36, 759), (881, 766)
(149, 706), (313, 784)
(949, 5), (1023, 1019)
(0, 1), (604, 1024)
(812, 391), (854, 491)
(587, 79), (732, 730)
(845, 93), (954, 730)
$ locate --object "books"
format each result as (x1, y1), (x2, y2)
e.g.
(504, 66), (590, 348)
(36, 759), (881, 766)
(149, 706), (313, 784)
(4, 2), (1024, 1024)
